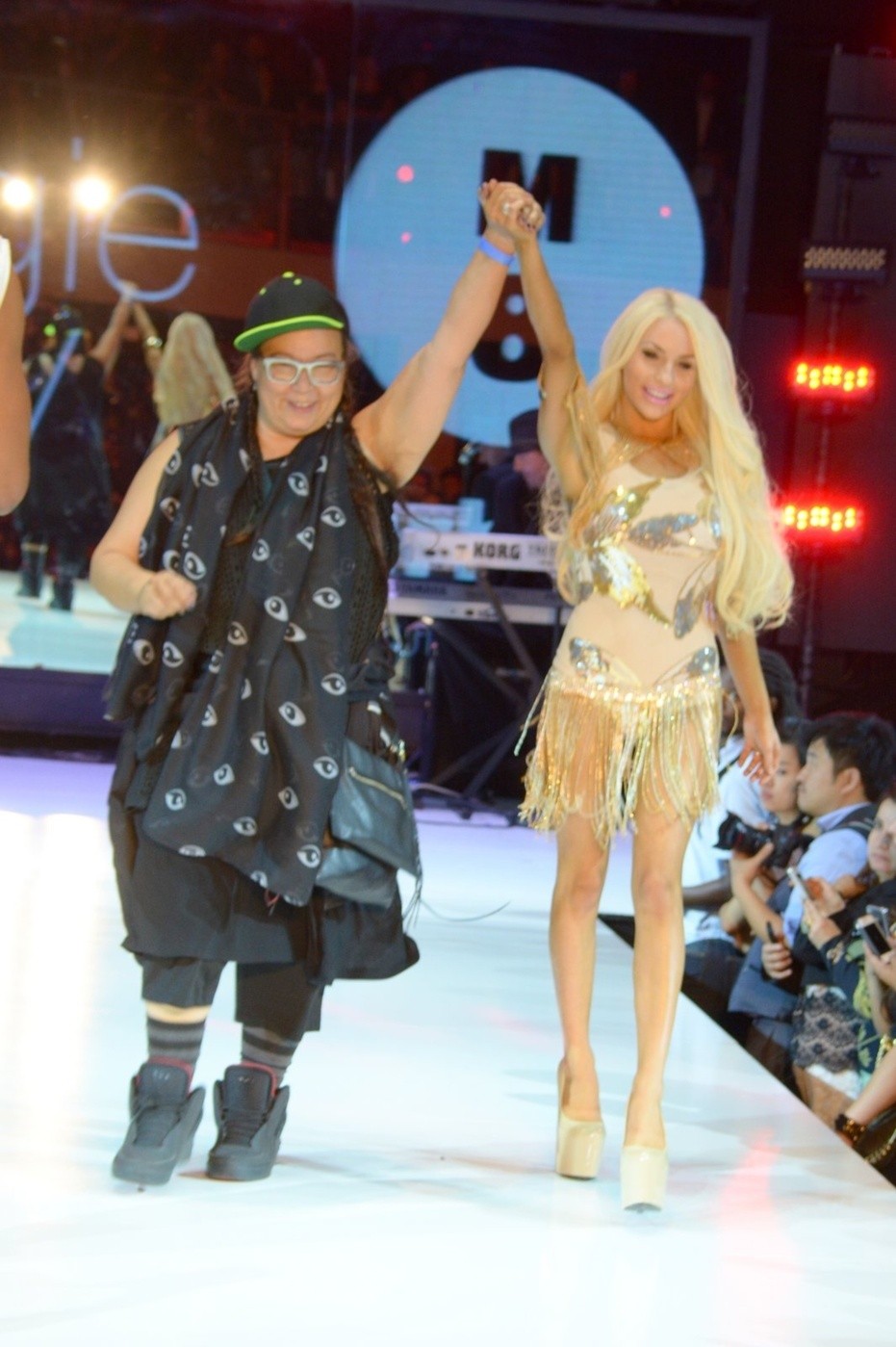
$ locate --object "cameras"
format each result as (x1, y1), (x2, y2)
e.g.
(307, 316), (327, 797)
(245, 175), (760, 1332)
(717, 811), (799, 870)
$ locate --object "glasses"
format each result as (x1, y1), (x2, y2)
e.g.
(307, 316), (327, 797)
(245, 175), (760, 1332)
(249, 356), (346, 384)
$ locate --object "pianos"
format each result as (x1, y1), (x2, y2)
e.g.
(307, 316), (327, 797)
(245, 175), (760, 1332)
(386, 527), (573, 828)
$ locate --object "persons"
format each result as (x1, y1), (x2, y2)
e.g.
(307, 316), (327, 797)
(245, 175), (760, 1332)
(498, 408), (553, 492)
(0, 234), (33, 517)
(678, 645), (895, 1184)
(122, 279), (241, 453)
(15, 284), (140, 614)
(86, 179), (548, 1184)
(483, 181), (794, 1211)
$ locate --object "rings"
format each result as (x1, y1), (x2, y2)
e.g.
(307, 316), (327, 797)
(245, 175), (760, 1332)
(501, 203), (511, 214)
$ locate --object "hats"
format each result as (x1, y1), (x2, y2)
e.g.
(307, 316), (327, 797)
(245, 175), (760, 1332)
(49, 302), (86, 332)
(508, 409), (539, 453)
(234, 272), (347, 354)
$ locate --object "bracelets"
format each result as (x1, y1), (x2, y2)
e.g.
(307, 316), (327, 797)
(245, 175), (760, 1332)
(477, 236), (517, 267)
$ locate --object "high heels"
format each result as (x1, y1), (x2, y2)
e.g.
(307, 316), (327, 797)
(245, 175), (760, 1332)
(620, 1141), (673, 1207)
(555, 1114), (606, 1178)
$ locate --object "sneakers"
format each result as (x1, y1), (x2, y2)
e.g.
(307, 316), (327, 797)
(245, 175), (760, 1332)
(16, 576), (42, 604)
(113, 1055), (205, 1185)
(208, 1064), (289, 1182)
(49, 584), (76, 611)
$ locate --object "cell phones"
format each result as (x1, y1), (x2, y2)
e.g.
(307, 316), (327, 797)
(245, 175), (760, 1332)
(785, 863), (811, 902)
(765, 921), (775, 945)
(859, 924), (890, 957)
